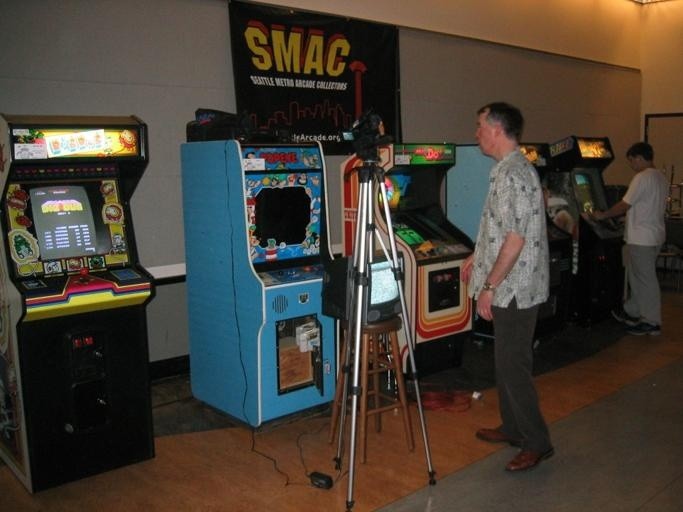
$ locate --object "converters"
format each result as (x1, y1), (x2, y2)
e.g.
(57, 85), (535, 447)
(309, 470), (333, 489)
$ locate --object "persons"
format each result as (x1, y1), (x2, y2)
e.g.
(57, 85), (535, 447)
(591, 142), (669, 337)
(458, 101), (555, 473)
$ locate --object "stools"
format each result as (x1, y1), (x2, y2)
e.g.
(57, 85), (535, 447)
(326, 315), (419, 469)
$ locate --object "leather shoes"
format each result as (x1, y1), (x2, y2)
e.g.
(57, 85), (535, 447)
(505, 446), (554, 472)
(476, 428), (522, 447)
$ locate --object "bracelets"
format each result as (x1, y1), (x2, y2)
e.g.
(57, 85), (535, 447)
(481, 282), (496, 292)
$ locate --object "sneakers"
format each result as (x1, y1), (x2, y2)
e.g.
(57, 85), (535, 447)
(628, 322), (661, 336)
(610, 308), (641, 326)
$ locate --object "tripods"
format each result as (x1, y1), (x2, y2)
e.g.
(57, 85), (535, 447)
(332, 164), (436, 511)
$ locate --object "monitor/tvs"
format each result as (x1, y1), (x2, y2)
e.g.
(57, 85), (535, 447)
(378, 172), (413, 212)
(254, 187), (311, 249)
(574, 174), (596, 214)
(19, 179), (113, 262)
